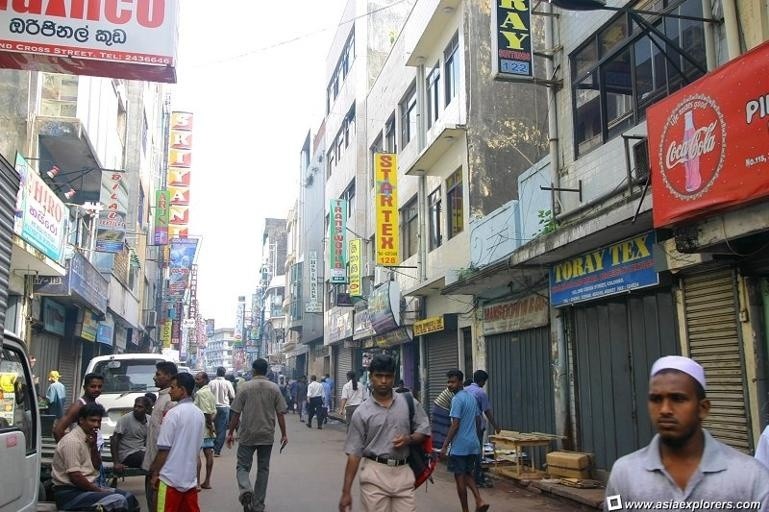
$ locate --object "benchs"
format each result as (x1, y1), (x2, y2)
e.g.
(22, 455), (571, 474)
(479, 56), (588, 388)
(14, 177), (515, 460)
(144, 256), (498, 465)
(39, 437), (115, 501)
(102, 468), (148, 487)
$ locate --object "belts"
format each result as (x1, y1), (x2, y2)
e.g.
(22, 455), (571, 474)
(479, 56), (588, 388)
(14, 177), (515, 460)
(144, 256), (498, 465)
(363, 454), (410, 468)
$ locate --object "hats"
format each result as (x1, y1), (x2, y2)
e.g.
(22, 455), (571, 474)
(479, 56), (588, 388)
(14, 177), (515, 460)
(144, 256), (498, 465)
(650, 356), (707, 390)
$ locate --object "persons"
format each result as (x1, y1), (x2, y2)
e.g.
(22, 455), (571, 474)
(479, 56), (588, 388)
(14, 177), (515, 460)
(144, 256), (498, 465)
(395, 368), (502, 512)
(25, 353), (157, 512)
(226, 357), (288, 511)
(150, 371), (206, 512)
(604, 355), (769, 512)
(145, 362), (176, 512)
(339, 355), (431, 511)
(754, 425), (769, 470)
(192, 365), (367, 492)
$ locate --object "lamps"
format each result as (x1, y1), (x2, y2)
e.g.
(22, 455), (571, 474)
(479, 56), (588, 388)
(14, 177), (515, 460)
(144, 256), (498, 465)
(10, 268), (39, 281)
(24, 157), (61, 180)
(47, 181), (78, 201)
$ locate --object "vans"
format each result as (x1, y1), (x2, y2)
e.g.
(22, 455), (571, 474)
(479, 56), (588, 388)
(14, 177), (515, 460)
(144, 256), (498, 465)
(79, 354), (177, 462)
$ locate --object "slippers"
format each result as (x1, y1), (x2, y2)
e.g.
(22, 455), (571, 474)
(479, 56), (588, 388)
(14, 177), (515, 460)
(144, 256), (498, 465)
(476, 504), (490, 512)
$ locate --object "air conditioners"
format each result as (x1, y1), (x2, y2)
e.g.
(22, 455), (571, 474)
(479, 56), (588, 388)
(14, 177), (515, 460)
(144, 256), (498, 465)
(145, 311), (158, 327)
(364, 261), (374, 277)
(373, 267), (391, 288)
(403, 297), (420, 326)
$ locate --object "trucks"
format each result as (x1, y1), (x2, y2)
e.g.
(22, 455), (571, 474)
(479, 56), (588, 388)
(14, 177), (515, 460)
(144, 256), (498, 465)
(0, 327), (41, 512)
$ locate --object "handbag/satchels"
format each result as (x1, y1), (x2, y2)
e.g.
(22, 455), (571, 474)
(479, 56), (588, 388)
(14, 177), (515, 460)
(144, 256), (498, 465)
(404, 392), (439, 494)
(318, 407), (328, 417)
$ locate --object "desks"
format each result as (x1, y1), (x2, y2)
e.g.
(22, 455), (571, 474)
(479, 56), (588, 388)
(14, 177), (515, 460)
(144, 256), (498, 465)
(488, 432), (556, 479)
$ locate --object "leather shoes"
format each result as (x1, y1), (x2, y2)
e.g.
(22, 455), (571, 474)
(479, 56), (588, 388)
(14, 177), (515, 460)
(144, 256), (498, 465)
(244, 492), (253, 512)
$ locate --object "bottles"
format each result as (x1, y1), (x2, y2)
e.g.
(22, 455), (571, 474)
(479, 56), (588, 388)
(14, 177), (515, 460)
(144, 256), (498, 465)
(683, 110), (701, 192)
